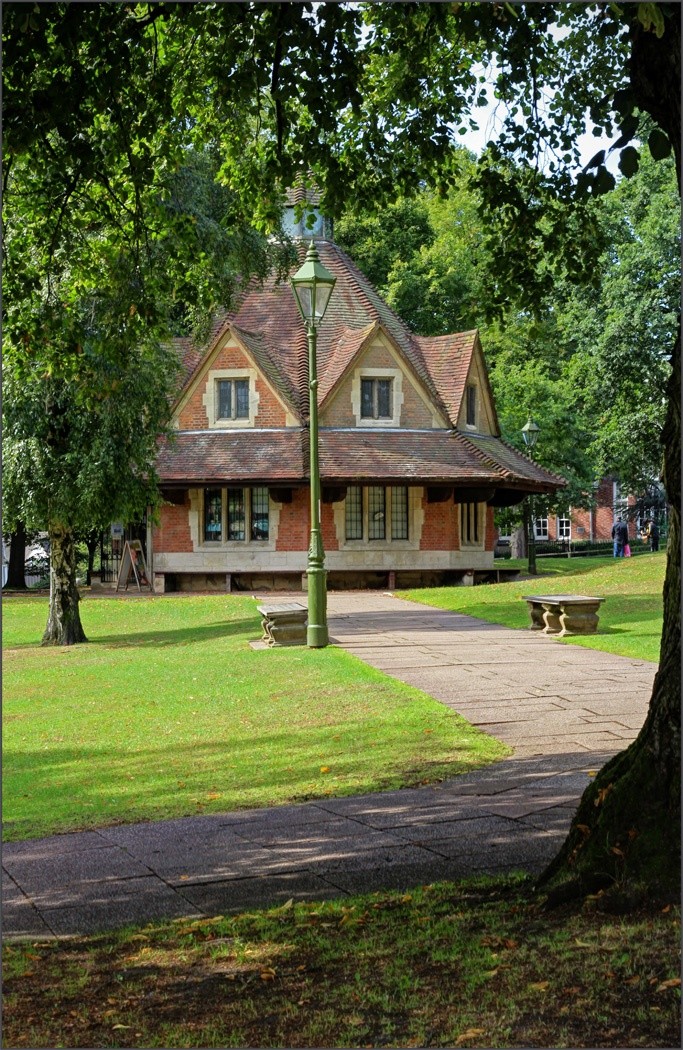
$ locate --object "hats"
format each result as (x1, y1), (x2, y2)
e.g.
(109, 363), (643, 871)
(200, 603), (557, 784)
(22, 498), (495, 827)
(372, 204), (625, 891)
(616, 516), (621, 519)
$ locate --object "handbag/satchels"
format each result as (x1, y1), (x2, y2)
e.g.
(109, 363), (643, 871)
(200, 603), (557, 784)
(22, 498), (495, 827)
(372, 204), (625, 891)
(624, 544), (630, 557)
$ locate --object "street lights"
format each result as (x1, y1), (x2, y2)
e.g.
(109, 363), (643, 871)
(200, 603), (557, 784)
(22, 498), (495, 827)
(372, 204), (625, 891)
(287, 237), (338, 652)
(520, 415), (540, 575)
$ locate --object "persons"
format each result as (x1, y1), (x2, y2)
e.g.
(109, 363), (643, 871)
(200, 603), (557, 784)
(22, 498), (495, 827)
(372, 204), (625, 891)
(611, 517), (628, 557)
(648, 520), (659, 551)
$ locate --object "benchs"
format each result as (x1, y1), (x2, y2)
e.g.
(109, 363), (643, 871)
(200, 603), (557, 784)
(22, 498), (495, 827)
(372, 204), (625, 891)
(522, 595), (607, 638)
(257, 603), (309, 648)
